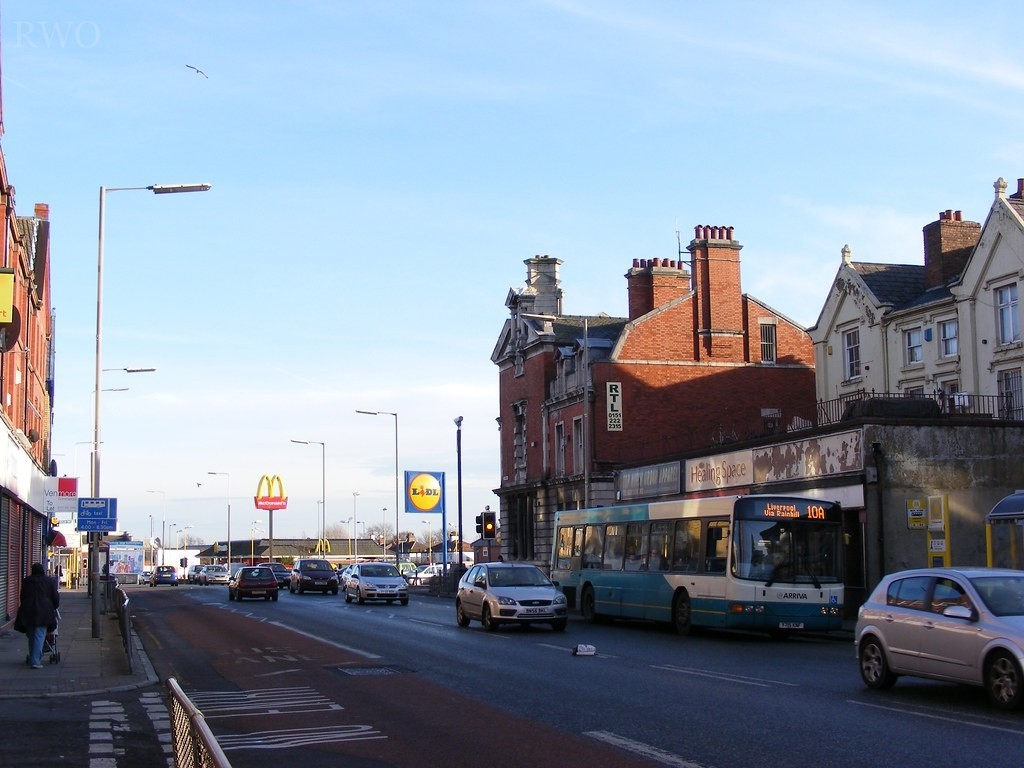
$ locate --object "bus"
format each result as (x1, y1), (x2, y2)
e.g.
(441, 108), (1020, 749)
(547, 493), (853, 644)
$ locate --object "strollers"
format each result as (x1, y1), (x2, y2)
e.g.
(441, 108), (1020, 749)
(26, 608), (61, 666)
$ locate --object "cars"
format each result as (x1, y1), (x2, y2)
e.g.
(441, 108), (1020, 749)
(148, 565), (178, 587)
(455, 561), (569, 633)
(251, 562), (291, 589)
(343, 562), (410, 607)
(289, 558), (339, 595)
(187, 562), (247, 586)
(138, 570), (152, 585)
(332, 560), (450, 593)
(227, 565), (281, 603)
(853, 565), (1024, 715)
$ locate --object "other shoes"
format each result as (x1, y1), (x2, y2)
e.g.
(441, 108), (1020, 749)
(33, 664), (44, 669)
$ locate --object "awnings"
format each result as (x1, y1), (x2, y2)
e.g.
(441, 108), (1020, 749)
(48, 530), (67, 547)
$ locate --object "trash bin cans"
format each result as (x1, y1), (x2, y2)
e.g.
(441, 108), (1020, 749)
(449, 563), (466, 593)
(71, 573), (79, 589)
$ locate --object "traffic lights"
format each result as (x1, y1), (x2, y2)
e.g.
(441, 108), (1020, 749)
(482, 511), (496, 540)
(476, 513), (483, 534)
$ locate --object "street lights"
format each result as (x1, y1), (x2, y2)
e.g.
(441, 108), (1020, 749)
(353, 492), (360, 564)
(449, 522), (457, 564)
(251, 520), (261, 566)
(290, 439), (327, 560)
(383, 507), (387, 562)
(453, 415), (465, 579)
(168, 523), (176, 550)
(86, 182), (213, 638)
(183, 526), (194, 550)
(356, 521), (365, 539)
(422, 520), (432, 572)
(176, 529), (184, 550)
(355, 410), (399, 568)
(340, 520), (351, 565)
(207, 471), (232, 578)
(317, 499), (324, 559)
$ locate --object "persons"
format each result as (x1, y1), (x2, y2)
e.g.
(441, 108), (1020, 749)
(650, 549), (668, 571)
(490, 573), (501, 585)
(20, 563), (59, 669)
(55, 563), (63, 577)
(114, 562), (130, 573)
(749, 551), (779, 578)
(498, 555), (503, 562)
